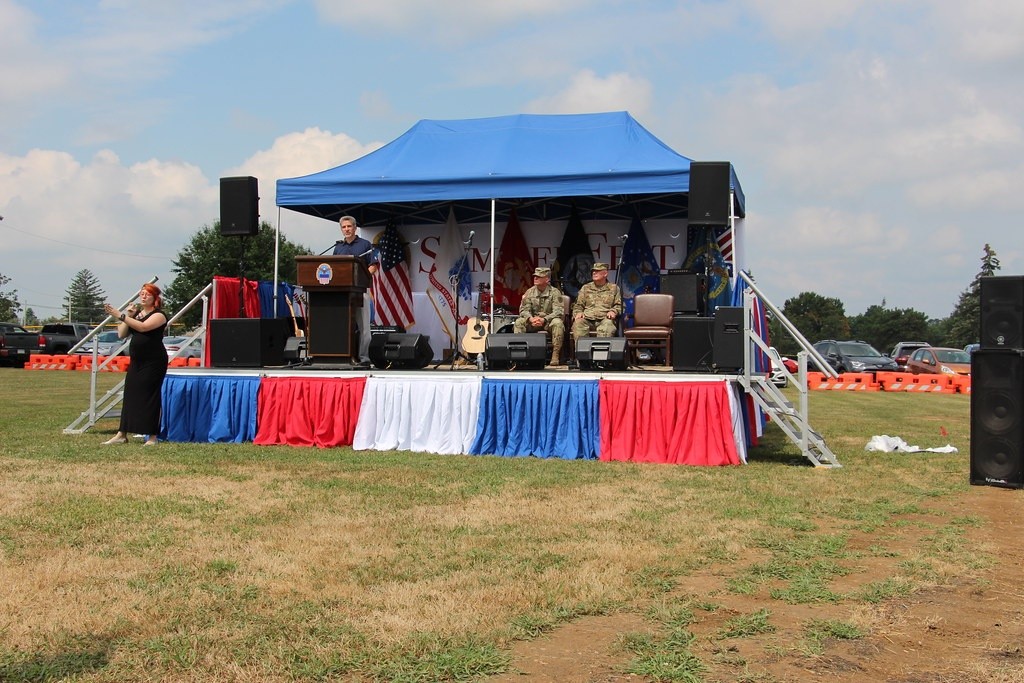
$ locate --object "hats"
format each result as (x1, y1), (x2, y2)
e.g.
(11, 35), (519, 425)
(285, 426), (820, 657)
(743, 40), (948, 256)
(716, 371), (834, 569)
(533, 267), (551, 277)
(591, 263), (609, 270)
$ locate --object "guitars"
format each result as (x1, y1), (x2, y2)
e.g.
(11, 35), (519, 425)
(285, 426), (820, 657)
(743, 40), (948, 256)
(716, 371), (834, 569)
(461, 282), (490, 353)
(285, 293), (314, 359)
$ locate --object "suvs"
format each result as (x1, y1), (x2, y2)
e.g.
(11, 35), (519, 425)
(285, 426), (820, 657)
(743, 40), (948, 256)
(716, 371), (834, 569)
(807, 340), (898, 374)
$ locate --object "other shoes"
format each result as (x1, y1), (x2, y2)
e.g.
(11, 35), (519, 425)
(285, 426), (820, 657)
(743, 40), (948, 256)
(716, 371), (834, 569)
(360, 362), (370, 370)
(100, 438), (128, 446)
(142, 441), (158, 447)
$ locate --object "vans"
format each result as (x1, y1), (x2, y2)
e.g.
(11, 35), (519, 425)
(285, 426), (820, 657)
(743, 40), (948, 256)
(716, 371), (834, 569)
(0, 323), (30, 367)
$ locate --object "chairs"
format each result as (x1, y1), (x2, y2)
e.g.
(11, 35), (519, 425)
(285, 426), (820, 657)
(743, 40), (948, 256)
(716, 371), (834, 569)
(6, 327), (15, 332)
(569, 317), (619, 362)
(528, 295), (570, 361)
(624, 294), (675, 366)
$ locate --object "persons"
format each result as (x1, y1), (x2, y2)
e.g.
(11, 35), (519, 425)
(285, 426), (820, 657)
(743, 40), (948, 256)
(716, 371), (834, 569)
(513, 267), (566, 366)
(332, 216), (379, 363)
(101, 284), (169, 447)
(571, 263), (621, 340)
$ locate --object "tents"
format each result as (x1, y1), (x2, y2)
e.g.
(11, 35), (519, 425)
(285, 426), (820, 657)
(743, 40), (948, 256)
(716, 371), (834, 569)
(269, 111), (749, 370)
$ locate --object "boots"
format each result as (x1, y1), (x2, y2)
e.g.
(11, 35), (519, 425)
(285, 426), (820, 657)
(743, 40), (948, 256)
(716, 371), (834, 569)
(550, 351), (559, 366)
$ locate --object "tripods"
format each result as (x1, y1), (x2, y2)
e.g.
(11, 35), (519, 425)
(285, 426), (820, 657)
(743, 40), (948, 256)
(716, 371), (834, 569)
(433, 240), (478, 372)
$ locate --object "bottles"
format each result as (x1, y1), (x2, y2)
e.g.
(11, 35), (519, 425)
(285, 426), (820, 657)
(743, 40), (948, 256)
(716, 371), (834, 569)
(476, 353), (484, 372)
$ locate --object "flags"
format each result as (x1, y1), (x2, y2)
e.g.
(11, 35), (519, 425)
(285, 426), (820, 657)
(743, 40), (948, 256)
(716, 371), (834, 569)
(426, 204), (471, 345)
(373, 213), (415, 332)
(614, 213), (661, 329)
(492, 210), (535, 317)
(550, 207), (597, 300)
(683, 225), (733, 313)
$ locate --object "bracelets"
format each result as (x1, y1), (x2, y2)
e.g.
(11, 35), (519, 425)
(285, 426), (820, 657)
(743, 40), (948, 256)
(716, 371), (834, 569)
(119, 313), (125, 321)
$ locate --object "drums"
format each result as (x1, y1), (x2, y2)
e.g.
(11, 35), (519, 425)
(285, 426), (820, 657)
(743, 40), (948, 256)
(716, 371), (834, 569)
(497, 323), (515, 335)
(494, 316), (512, 334)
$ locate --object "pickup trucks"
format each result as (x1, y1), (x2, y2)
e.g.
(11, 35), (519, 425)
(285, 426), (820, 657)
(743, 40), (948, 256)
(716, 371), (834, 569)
(0, 322), (89, 368)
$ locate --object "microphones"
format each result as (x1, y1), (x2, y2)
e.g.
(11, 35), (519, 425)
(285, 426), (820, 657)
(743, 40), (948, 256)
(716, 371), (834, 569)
(469, 231), (475, 240)
(339, 240), (345, 245)
(357, 248), (375, 257)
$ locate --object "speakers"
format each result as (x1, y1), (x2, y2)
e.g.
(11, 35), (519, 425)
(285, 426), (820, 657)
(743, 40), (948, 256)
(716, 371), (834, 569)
(284, 336), (312, 364)
(687, 161), (730, 229)
(969, 349), (1024, 489)
(485, 333), (547, 370)
(576, 337), (630, 371)
(660, 273), (706, 313)
(712, 306), (745, 369)
(979, 275), (1024, 350)
(369, 333), (434, 371)
(210, 318), (289, 368)
(220, 176), (260, 237)
(672, 317), (715, 372)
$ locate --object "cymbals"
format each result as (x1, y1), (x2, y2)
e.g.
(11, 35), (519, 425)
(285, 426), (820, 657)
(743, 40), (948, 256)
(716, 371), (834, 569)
(492, 303), (515, 311)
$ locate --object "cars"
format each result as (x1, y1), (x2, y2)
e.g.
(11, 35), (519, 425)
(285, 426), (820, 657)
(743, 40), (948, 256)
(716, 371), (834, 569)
(905, 347), (971, 376)
(782, 357), (798, 374)
(163, 337), (202, 363)
(770, 347), (788, 388)
(75, 332), (132, 357)
(964, 344), (980, 355)
(890, 342), (933, 372)
